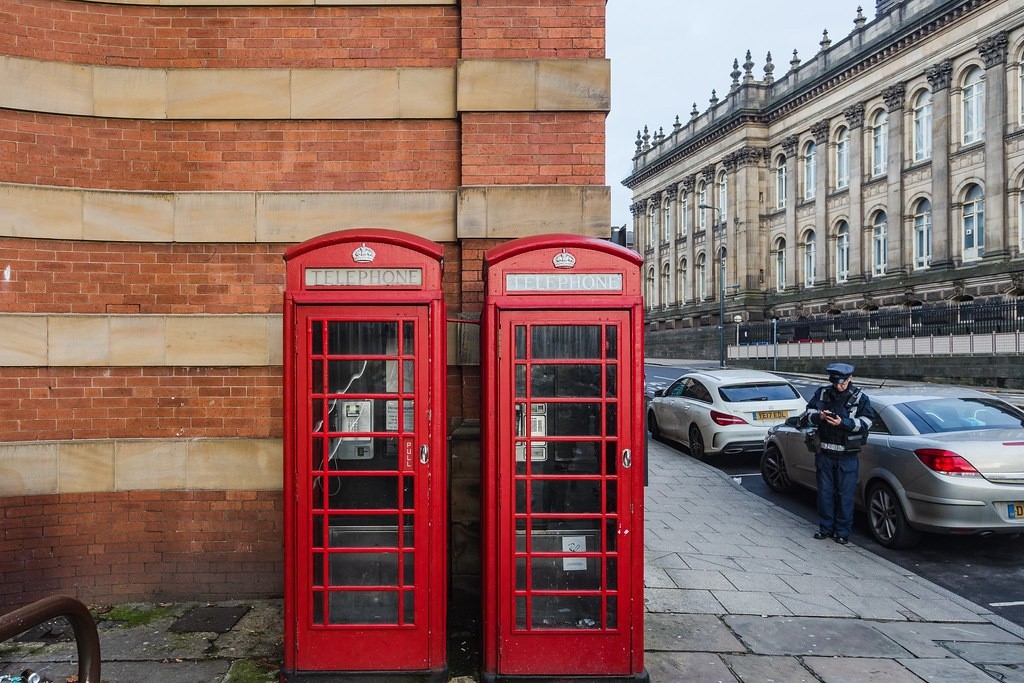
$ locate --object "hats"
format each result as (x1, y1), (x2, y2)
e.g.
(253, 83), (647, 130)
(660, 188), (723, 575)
(826, 362), (855, 384)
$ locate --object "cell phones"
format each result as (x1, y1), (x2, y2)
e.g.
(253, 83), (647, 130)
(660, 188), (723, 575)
(826, 412), (837, 420)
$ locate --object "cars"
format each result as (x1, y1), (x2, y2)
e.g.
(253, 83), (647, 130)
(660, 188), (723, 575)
(648, 371), (811, 460)
(760, 384), (1023, 551)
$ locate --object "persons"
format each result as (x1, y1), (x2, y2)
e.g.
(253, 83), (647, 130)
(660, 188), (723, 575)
(800, 363), (874, 543)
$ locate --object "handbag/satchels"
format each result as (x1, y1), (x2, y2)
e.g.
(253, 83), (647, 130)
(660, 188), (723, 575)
(804, 428), (821, 453)
(845, 434), (863, 453)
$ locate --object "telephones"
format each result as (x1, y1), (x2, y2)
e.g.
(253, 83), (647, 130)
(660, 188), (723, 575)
(515, 403), (547, 463)
(327, 401), (372, 459)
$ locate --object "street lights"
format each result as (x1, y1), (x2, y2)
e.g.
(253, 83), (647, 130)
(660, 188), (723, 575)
(699, 204), (725, 367)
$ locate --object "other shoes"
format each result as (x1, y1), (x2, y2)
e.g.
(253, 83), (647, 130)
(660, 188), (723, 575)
(835, 532), (848, 544)
(814, 530), (832, 539)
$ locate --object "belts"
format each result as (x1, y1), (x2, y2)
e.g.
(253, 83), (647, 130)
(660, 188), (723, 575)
(821, 442), (845, 451)
